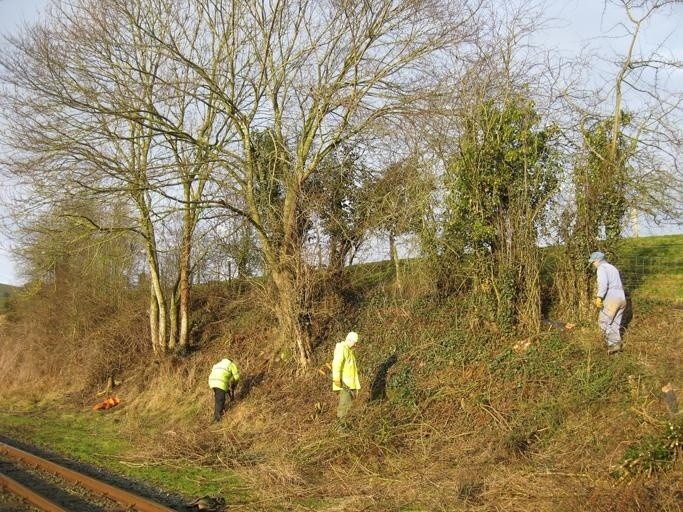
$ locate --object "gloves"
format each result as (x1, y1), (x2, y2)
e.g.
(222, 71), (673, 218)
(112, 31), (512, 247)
(596, 298), (604, 309)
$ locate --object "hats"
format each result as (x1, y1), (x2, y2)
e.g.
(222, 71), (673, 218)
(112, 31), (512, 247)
(589, 252), (605, 263)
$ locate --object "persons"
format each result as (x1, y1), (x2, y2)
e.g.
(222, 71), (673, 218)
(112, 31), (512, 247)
(207, 355), (240, 422)
(586, 250), (628, 357)
(328, 331), (362, 423)
